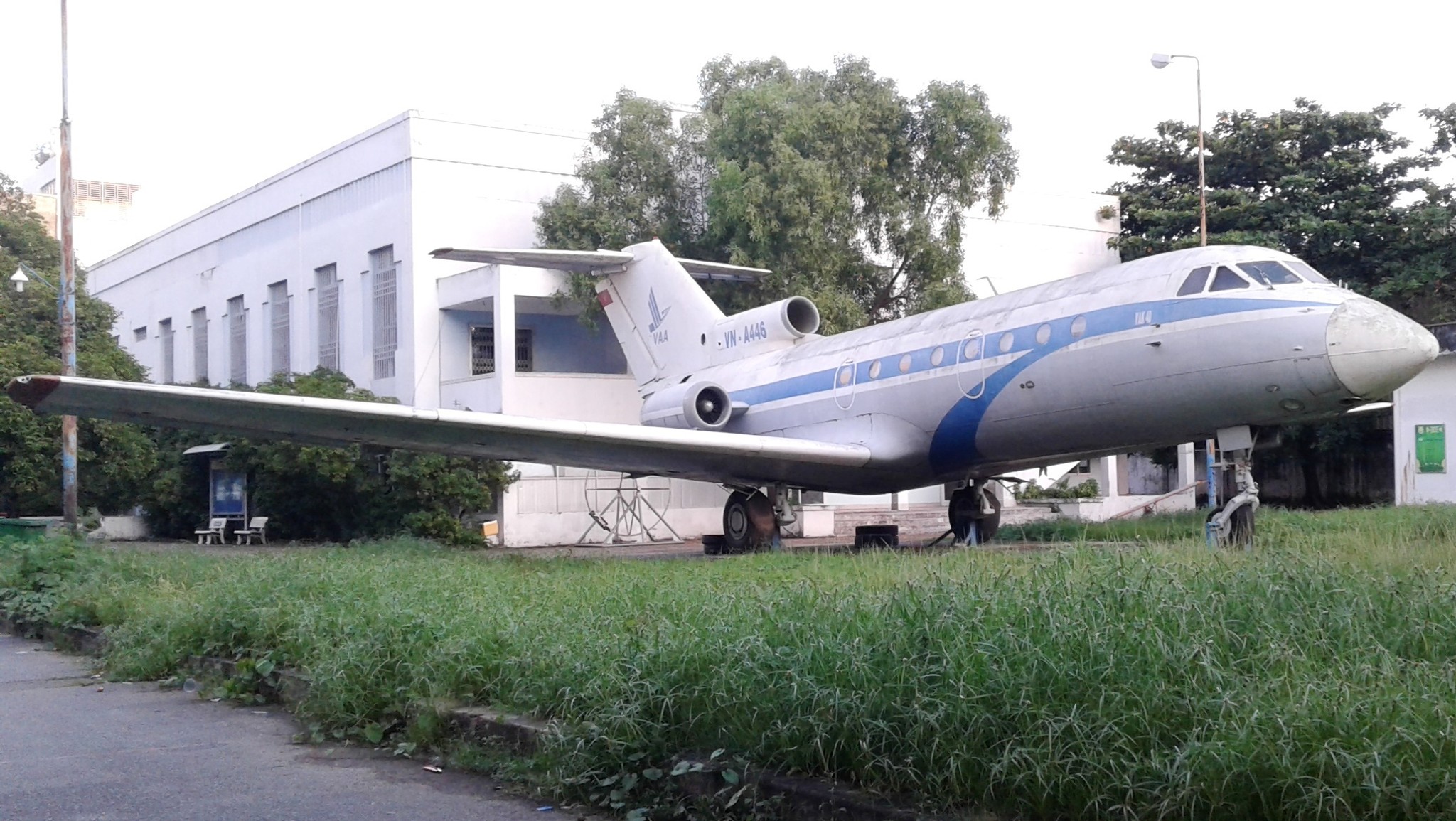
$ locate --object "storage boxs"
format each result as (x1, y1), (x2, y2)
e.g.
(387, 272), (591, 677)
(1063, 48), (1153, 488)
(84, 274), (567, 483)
(478, 520), (499, 535)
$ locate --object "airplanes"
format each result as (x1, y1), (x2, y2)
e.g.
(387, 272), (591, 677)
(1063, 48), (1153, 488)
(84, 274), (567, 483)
(3, 234), (1444, 553)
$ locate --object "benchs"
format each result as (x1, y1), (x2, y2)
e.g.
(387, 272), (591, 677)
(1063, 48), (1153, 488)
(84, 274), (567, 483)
(234, 517), (269, 545)
(194, 518), (227, 545)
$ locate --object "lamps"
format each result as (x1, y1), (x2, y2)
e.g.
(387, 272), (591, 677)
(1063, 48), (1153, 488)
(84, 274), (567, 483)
(10, 267), (28, 292)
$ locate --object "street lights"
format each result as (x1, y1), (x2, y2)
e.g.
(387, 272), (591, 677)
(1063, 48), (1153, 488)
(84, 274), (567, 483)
(1148, 53), (1217, 511)
